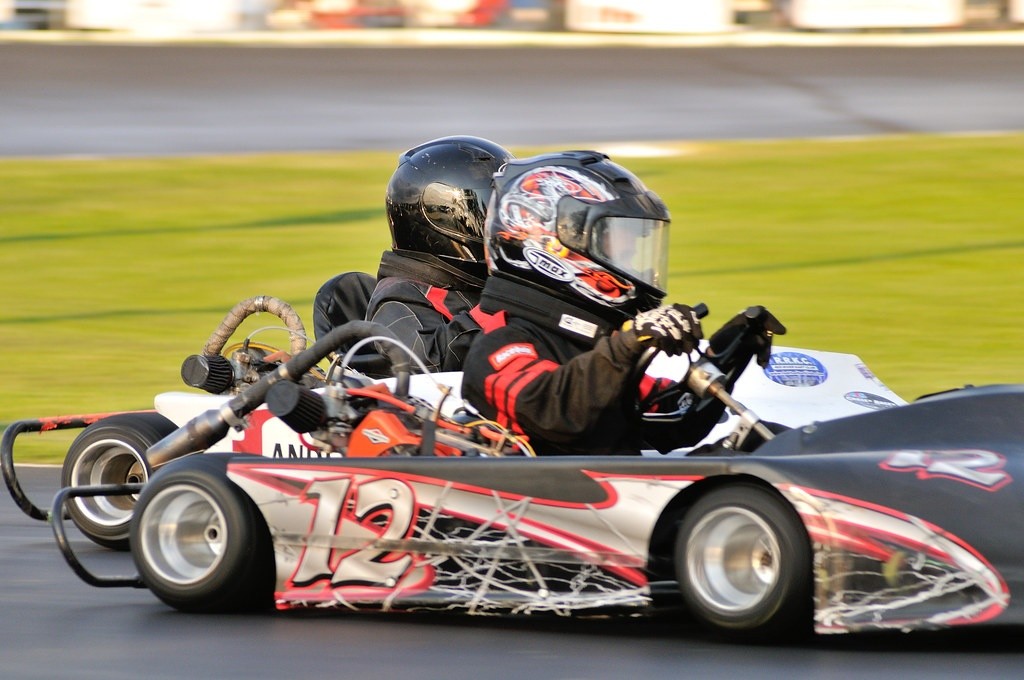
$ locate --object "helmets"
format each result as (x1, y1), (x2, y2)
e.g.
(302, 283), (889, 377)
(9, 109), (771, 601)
(384, 135), (518, 266)
(482, 148), (672, 321)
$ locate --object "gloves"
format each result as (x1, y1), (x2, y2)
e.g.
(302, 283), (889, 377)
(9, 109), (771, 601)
(635, 302), (704, 357)
(708, 305), (787, 368)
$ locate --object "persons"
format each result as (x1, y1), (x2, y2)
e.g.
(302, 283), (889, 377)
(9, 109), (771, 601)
(460, 150), (786, 460)
(364, 136), (517, 377)
